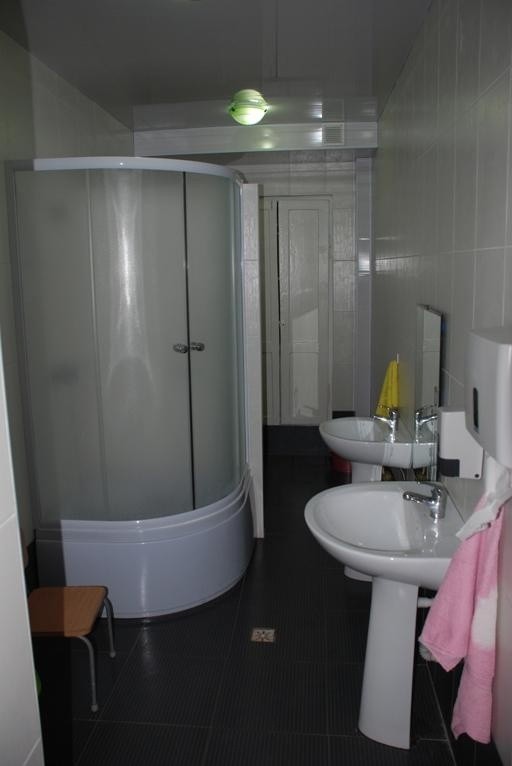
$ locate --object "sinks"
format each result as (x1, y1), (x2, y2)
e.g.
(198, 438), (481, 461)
(304, 481), (465, 593)
(319, 417), (413, 470)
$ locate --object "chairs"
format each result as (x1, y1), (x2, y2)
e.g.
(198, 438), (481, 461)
(21, 540), (116, 716)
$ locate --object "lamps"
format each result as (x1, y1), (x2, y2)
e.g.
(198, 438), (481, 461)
(225, 89), (269, 124)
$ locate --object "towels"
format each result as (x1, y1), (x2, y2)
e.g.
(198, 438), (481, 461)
(411, 456), (512, 747)
(375, 359), (399, 418)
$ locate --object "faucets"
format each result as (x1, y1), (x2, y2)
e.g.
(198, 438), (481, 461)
(402, 479), (448, 518)
(415, 405), (439, 433)
(373, 405), (400, 432)
